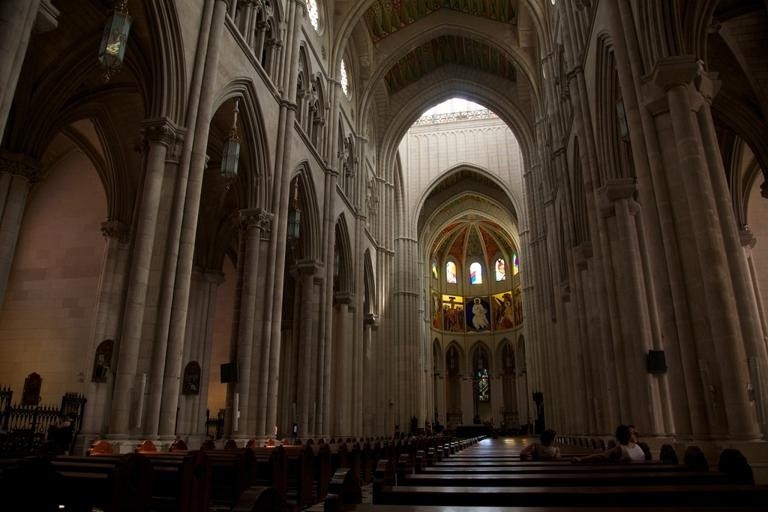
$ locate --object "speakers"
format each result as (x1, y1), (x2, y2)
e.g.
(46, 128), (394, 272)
(649, 349), (666, 374)
(220, 362), (239, 383)
(533, 392), (543, 402)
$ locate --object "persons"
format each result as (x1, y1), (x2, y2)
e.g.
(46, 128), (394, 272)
(519, 428), (561, 460)
(571, 424), (646, 463)
(95, 343), (117, 383)
(627, 426), (652, 460)
(472, 297), (490, 331)
(41, 411), (77, 456)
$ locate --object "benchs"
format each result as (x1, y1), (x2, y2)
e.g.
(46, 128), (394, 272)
(1, 426), (768, 512)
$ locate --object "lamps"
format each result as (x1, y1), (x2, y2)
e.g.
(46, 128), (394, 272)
(288, 177), (302, 244)
(221, 98), (242, 195)
(96, 0), (135, 83)
